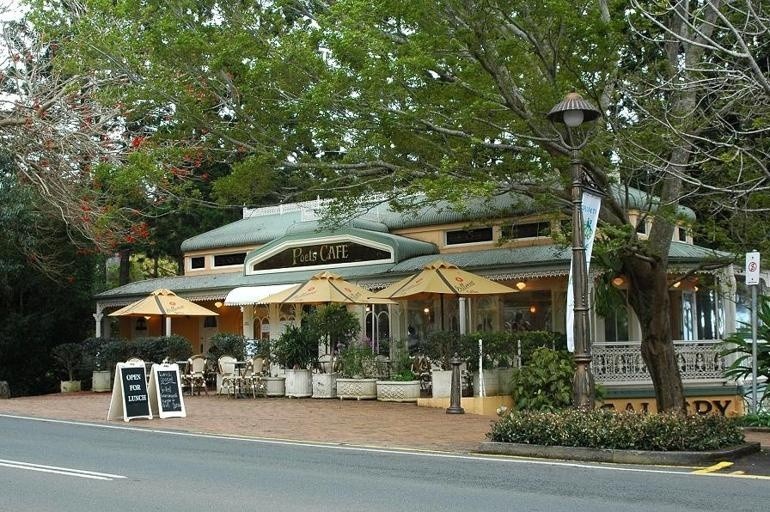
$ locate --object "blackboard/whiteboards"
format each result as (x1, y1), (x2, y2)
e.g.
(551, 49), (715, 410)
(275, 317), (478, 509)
(154, 367), (187, 419)
(119, 365), (154, 423)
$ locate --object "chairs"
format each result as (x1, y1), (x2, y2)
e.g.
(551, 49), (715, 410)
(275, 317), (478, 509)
(125, 357), (148, 364)
(164, 355), (266, 401)
(409, 350), (476, 396)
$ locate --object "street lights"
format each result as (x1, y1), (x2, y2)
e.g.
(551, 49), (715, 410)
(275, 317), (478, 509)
(547, 92), (603, 411)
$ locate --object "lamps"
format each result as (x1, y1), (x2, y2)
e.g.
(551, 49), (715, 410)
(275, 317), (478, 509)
(213, 299), (224, 308)
(612, 275), (625, 287)
(143, 314), (152, 320)
(516, 280), (529, 290)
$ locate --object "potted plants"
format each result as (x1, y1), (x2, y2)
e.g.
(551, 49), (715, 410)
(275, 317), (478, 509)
(38, 329), (192, 395)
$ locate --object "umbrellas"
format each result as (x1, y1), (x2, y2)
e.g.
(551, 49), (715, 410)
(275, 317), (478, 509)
(104, 285), (220, 338)
(253, 267), (399, 355)
(366, 257), (520, 362)
